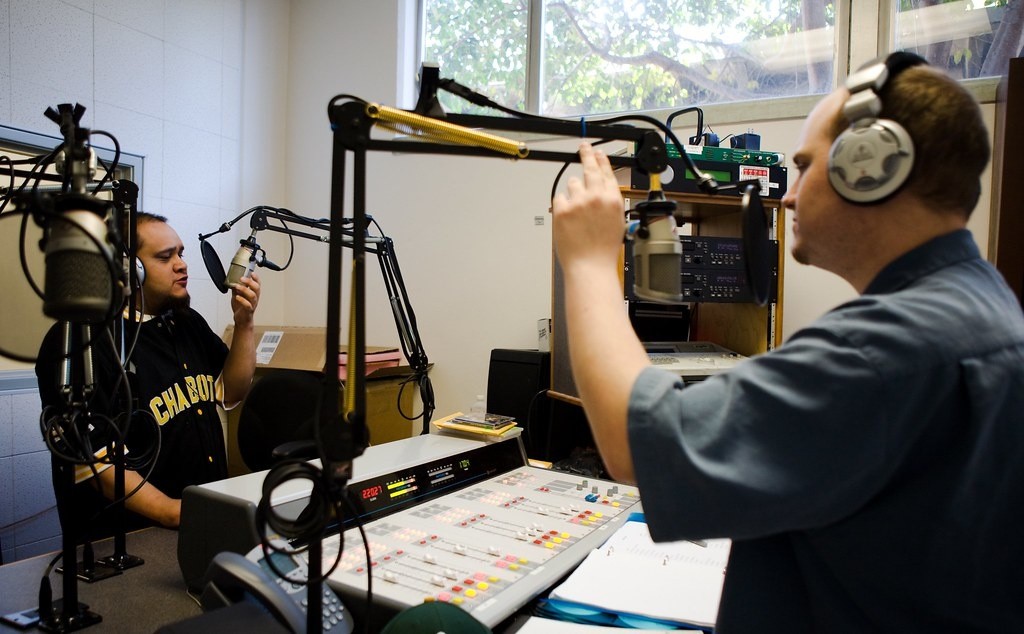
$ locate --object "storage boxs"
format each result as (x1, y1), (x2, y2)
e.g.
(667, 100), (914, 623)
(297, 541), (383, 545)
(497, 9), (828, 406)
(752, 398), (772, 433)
(220, 324), (416, 477)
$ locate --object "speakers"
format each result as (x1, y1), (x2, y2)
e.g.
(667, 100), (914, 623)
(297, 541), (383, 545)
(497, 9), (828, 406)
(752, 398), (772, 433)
(485, 347), (554, 461)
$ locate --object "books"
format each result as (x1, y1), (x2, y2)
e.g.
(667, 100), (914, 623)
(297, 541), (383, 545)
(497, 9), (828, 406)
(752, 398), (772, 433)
(551, 521), (733, 629)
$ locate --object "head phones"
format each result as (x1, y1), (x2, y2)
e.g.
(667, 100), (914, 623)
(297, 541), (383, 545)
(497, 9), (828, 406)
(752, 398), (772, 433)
(827, 50), (930, 205)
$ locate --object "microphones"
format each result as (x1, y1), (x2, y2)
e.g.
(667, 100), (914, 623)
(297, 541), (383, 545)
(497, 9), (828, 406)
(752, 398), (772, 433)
(41, 176), (115, 325)
(633, 190), (682, 304)
(225, 235), (256, 289)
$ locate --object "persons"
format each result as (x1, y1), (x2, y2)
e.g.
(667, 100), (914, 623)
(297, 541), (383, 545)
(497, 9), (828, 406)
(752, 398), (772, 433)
(548, 55), (1023, 634)
(35, 212), (261, 545)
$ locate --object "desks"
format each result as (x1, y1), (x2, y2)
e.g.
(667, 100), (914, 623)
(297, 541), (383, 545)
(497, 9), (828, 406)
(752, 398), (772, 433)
(0, 524), (208, 634)
